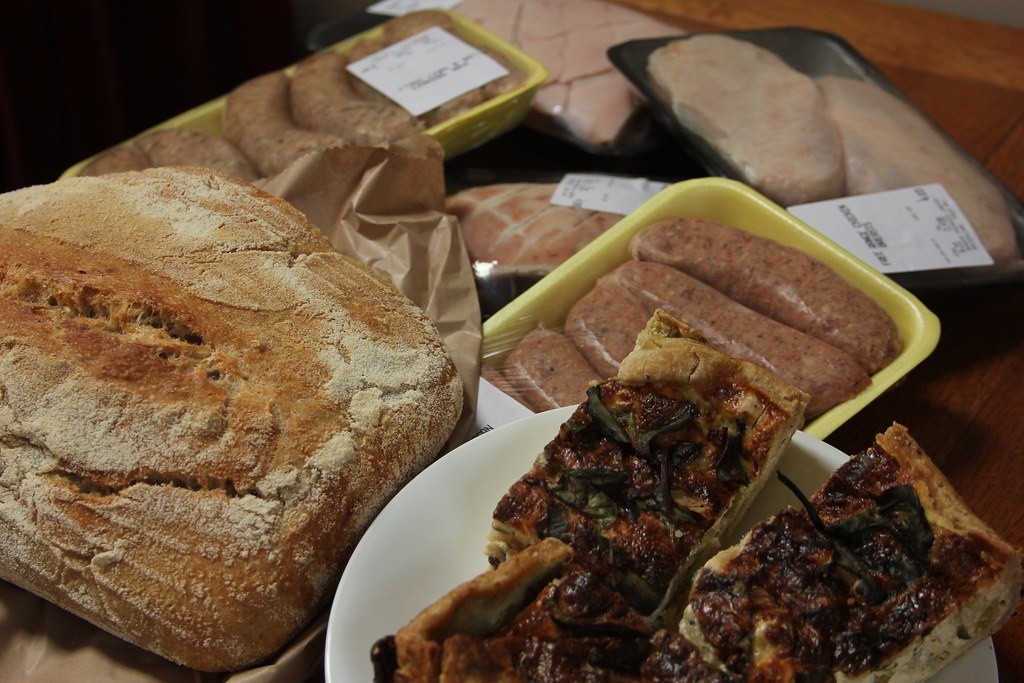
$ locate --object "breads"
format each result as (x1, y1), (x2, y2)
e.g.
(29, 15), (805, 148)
(0, 168), (465, 671)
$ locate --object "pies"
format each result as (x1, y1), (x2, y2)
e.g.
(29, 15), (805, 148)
(369, 310), (1024, 683)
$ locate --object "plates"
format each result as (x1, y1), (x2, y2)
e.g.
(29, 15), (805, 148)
(606, 25), (1020, 286)
(59, 7), (546, 256)
(324, 400), (998, 683)
(480, 176), (941, 441)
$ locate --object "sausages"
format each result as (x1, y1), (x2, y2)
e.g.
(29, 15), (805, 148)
(504, 218), (902, 435)
(74, 9), (524, 186)
